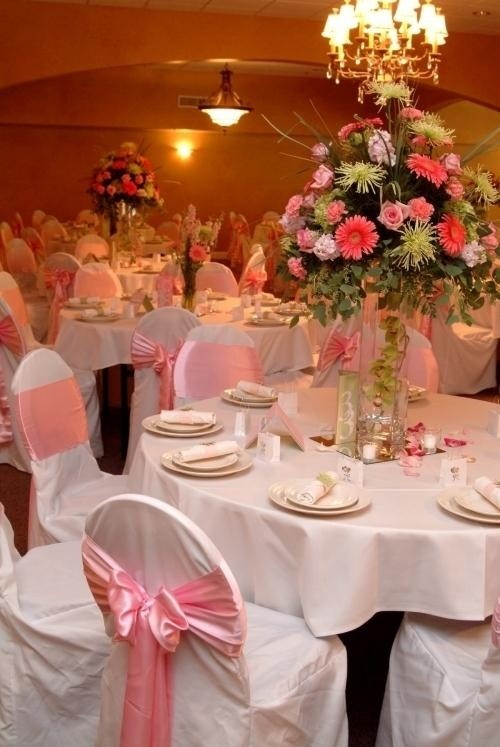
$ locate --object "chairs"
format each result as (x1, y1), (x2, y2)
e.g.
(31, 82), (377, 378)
(0, 210), (500, 747)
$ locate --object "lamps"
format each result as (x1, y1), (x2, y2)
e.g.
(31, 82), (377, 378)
(199, 63), (252, 126)
(321, 0), (449, 84)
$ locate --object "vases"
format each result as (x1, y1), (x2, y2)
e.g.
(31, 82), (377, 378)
(117, 201), (132, 233)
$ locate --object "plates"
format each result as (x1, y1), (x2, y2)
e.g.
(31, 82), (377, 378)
(253, 298), (281, 305)
(268, 479), (374, 518)
(247, 311), (288, 325)
(434, 481), (500, 527)
(272, 306), (312, 316)
(73, 312), (123, 324)
(64, 301), (106, 309)
(141, 412), (223, 436)
(135, 270), (161, 274)
(220, 389), (279, 408)
(208, 291), (226, 300)
(162, 450), (253, 476)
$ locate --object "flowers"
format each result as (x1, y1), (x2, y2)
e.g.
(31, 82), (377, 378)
(259, 81), (500, 406)
(88, 138), (168, 209)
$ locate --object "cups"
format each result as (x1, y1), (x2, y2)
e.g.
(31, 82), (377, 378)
(360, 413), (465, 493)
(122, 303), (134, 319)
(152, 252), (161, 269)
